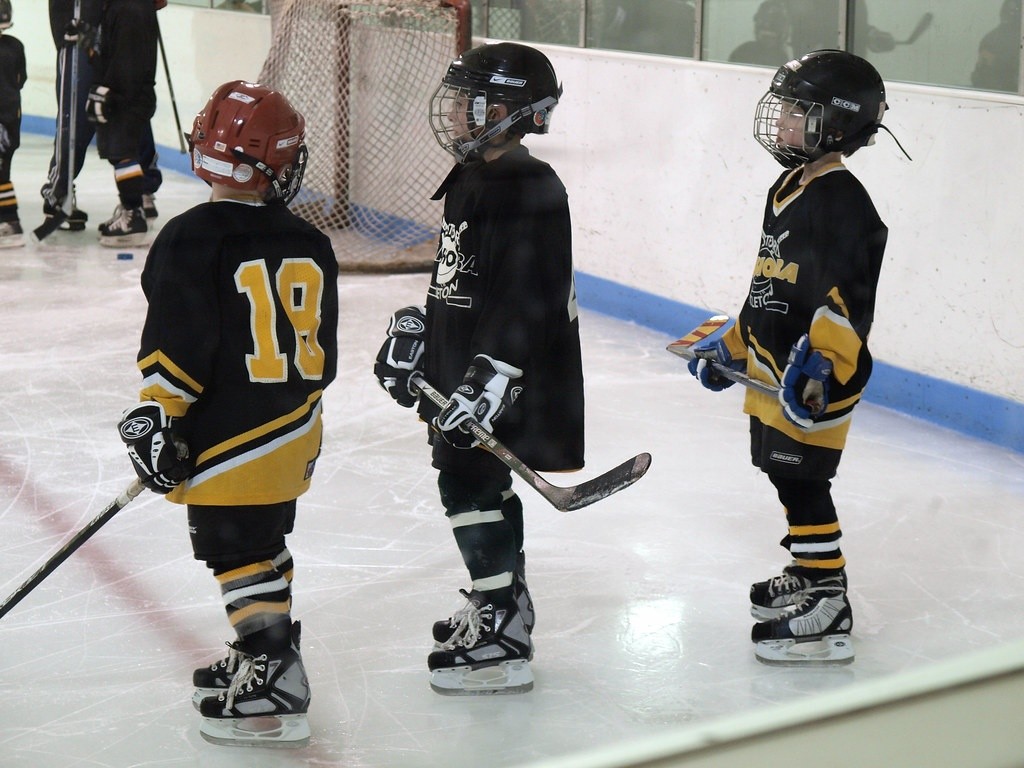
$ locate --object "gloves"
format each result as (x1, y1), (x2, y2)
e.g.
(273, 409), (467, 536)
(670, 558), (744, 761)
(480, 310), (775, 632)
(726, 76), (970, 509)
(373, 304), (426, 408)
(688, 338), (747, 391)
(429, 354), (524, 450)
(0, 122), (11, 153)
(84, 85), (114, 125)
(117, 401), (198, 495)
(778, 334), (833, 432)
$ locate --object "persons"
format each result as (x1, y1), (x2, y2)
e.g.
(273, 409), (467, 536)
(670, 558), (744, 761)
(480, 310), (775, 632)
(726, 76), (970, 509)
(666, 47), (912, 667)
(40, 0), (167, 247)
(520, 0), (1024, 99)
(117, 79), (339, 749)
(0, 0), (27, 249)
(371, 42), (586, 695)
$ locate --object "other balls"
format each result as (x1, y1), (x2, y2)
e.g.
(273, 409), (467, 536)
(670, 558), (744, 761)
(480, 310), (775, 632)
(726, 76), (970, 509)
(117, 253), (133, 259)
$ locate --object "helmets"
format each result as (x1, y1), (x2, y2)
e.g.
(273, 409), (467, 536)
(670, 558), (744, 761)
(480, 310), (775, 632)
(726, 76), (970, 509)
(184, 80), (309, 207)
(753, 49), (886, 171)
(428, 42), (560, 165)
(0, 0), (14, 33)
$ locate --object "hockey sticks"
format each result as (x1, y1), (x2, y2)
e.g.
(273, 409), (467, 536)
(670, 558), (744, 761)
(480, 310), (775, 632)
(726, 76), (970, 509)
(0, 443), (190, 618)
(29, 0), (82, 245)
(156, 10), (188, 156)
(664, 311), (780, 398)
(412, 373), (653, 515)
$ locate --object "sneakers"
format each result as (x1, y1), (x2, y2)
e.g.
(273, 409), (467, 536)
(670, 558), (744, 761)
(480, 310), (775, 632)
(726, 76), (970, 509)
(428, 573), (535, 689)
(750, 582), (855, 662)
(96, 204), (126, 240)
(197, 618), (310, 741)
(43, 199), (88, 231)
(432, 549), (536, 662)
(142, 190), (159, 231)
(193, 620), (302, 704)
(749, 560), (848, 619)
(100, 208), (149, 247)
(0, 221), (26, 248)
